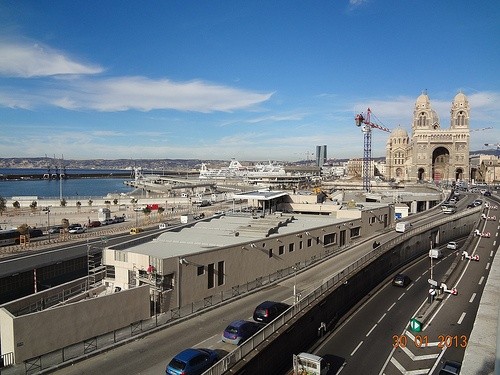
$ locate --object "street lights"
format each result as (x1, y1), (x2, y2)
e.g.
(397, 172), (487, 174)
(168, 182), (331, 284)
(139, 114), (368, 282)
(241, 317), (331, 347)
(42, 207), (51, 244)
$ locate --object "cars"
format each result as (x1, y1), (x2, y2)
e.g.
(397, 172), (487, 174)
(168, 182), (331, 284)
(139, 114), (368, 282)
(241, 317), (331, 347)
(392, 274), (411, 288)
(441, 184), (491, 215)
(103, 217), (124, 225)
(89, 221), (101, 227)
(447, 241), (459, 250)
(69, 226), (85, 234)
(222, 320), (258, 346)
(439, 359), (462, 375)
(69, 224), (81, 229)
(165, 347), (219, 375)
(253, 300), (290, 324)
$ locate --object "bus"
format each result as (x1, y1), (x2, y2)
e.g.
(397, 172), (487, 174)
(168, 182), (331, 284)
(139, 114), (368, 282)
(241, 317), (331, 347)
(0, 227), (31, 247)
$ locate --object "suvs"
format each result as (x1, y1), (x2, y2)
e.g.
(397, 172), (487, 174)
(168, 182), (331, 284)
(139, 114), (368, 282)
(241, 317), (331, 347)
(48, 225), (63, 234)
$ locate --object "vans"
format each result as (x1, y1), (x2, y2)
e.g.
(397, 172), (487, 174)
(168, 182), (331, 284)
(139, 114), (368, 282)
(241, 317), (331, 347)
(428, 248), (443, 261)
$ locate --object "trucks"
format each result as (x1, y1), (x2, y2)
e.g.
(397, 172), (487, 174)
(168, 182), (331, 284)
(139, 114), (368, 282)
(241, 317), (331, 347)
(292, 351), (330, 375)
(396, 221), (413, 233)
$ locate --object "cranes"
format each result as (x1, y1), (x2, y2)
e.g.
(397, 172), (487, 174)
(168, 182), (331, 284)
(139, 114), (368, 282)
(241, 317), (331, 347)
(354, 107), (392, 193)
